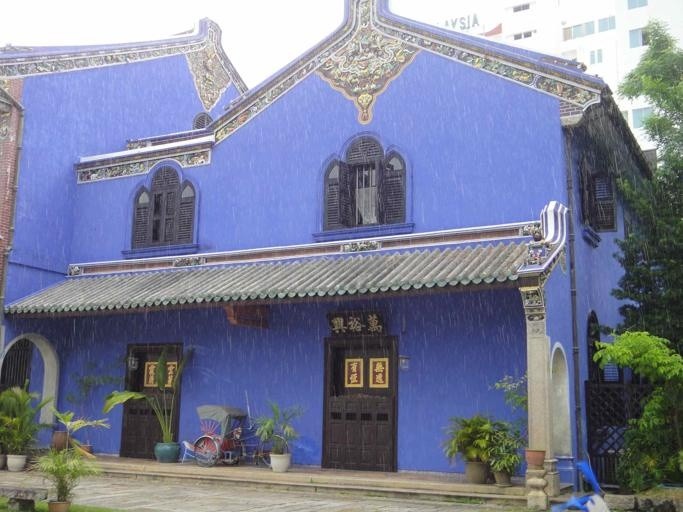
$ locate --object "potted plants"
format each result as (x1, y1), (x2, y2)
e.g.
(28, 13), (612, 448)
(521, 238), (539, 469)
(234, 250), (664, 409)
(0, 341), (197, 512)
(440, 414), (523, 487)
(253, 397), (307, 473)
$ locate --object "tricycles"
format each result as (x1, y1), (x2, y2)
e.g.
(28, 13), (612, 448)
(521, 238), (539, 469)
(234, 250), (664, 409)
(180, 403), (290, 469)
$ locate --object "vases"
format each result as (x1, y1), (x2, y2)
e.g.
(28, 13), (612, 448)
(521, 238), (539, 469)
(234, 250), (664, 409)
(525, 448), (546, 466)
